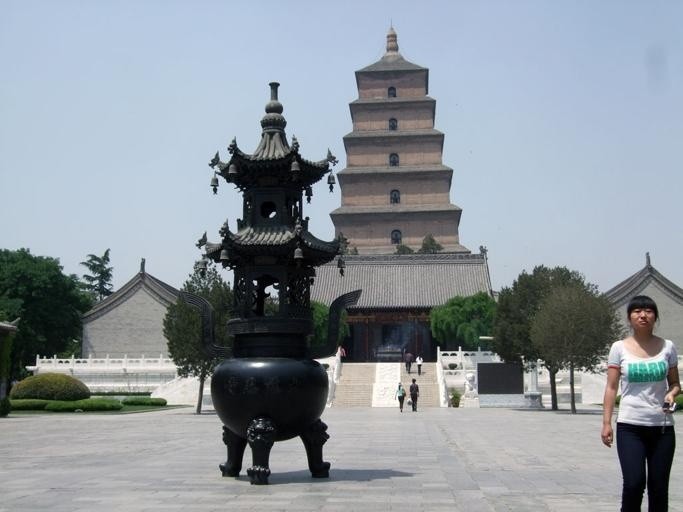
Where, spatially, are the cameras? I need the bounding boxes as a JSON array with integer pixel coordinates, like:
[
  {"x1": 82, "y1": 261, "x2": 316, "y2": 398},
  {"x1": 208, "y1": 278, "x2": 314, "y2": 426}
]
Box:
[{"x1": 662, "y1": 401, "x2": 678, "y2": 412}]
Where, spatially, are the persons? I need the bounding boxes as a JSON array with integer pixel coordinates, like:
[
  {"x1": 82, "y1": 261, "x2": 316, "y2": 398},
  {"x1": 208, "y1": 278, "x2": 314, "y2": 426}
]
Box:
[
  {"x1": 600, "y1": 295, "x2": 681, "y2": 512},
  {"x1": 394, "y1": 383, "x2": 406, "y2": 412},
  {"x1": 340, "y1": 346, "x2": 346, "y2": 362},
  {"x1": 415, "y1": 354, "x2": 423, "y2": 375},
  {"x1": 403, "y1": 351, "x2": 413, "y2": 374},
  {"x1": 409, "y1": 378, "x2": 419, "y2": 411}
]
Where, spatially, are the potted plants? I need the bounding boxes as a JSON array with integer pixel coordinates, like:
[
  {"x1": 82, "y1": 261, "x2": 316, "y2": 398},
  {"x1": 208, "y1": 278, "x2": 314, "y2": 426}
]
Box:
[{"x1": 451, "y1": 393, "x2": 459, "y2": 407}]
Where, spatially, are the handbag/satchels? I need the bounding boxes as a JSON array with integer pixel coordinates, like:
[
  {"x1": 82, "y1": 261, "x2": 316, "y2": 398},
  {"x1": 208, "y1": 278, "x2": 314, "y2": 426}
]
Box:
[
  {"x1": 402, "y1": 389, "x2": 406, "y2": 397},
  {"x1": 407, "y1": 398, "x2": 413, "y2": 405}
]
